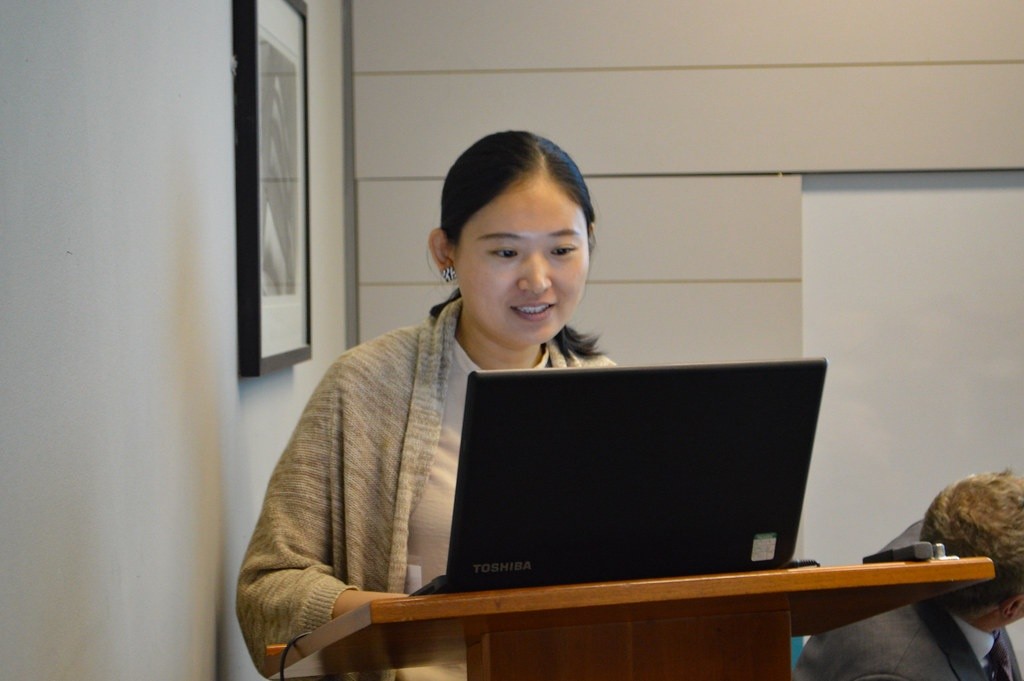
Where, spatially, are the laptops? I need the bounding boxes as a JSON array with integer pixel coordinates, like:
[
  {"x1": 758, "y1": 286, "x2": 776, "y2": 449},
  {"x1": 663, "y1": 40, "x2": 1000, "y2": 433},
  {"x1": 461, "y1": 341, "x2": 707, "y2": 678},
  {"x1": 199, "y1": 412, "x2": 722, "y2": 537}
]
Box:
[{"x1": 410, "y1": 356, "x2": 831, "y2": 596}]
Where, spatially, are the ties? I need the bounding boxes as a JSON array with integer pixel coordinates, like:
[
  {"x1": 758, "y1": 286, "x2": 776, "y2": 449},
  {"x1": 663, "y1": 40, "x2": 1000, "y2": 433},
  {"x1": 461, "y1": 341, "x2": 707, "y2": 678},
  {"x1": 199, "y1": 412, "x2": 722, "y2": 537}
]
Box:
[{"x1": 986, "y1": 629, "x2": 1013, "y2": 681}]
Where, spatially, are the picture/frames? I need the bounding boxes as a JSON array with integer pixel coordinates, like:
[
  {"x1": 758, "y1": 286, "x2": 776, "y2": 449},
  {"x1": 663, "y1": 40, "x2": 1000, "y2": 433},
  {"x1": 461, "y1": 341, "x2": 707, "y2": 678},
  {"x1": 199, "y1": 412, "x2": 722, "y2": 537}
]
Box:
[{"x1": 230, "y1": 1, "x2": 316, "y2": 377}]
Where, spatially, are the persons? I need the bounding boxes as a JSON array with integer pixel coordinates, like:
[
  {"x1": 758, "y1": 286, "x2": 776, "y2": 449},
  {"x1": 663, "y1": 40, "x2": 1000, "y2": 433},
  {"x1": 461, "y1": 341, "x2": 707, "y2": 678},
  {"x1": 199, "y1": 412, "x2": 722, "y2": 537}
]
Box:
[
  {"x1": 235, "y1": 130, "x2": 620, "y2": 681},
  {"x1": 791, "y1": 466, "x2": 1024, "y2": 681}
]
